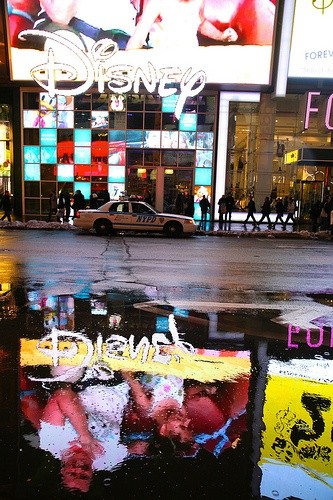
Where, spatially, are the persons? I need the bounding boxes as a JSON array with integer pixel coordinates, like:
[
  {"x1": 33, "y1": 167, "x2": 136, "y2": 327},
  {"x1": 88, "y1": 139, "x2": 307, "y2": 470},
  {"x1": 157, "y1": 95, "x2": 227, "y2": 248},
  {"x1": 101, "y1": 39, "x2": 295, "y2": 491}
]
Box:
[
  {"x1": 63, "y1": 192, "x2": 70, "y2": 222},
  {"x1": 198, "y1": 195, "x2": 210, "y2": 224},
  {"x1": 142, "y1": 190, "x2": 150, "y2": 205},
  {"x1": 56, "y1": 193, "x2": 64, "y2": 222},
  {"x1": 217, "y1": 195, "x2": 226, "y2": 223},
  {"x1": 70, "y1": 190, "x2": 109, "y2": 219},
  {"x1": 184, "y1": 195, "x2": 194, "y2": 218},
  {"x1": 224, "y1": 192, "x2": 234, "y2": 222},
  {"x1": 0, "y1": 191, "x2": 12, "y2": 223},
  {"x1": 272, "y1": 198, "x2": 285, "y2": 228},
  {"x1": 255, "y1": 197, "x2": 272, "y2": 226},
  {"x1": 282, "y1": 197, "x2": 297, "y2": 227},
  {"x1": 309, "y1": 194, "x2": 333, "y2": 236},
  {"x1": 32, "y1": 0, "x2": 142, "y2": 56},
  {"x1": 120, "y1": 369, "x2": 216, "y2": 455},
  {"x1": 241, "y1": 198, "x2": 256, "y2": 224},
  {"x1": 125, "y1": 0, "x2": 238, "y2": 51},
  {"x1": 37, "y1": 366, "x2": 129, "y2": 496},
  {"x1": 270, "y1": 189, "x2": 276, "y2": 201},
  {"x1": 45, "y1": 193, "x2": 60, "y2": 222},
  {"x1": 174, "y1": 194, "x2": 183, "y2": 214}
]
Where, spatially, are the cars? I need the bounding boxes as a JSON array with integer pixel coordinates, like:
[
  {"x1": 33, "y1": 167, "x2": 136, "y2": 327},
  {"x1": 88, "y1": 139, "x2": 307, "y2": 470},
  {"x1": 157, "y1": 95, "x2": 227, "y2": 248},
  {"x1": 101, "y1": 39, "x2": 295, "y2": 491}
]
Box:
[{"x1": 73, "y1": 195, "x2": 195, "y2": 238}]
